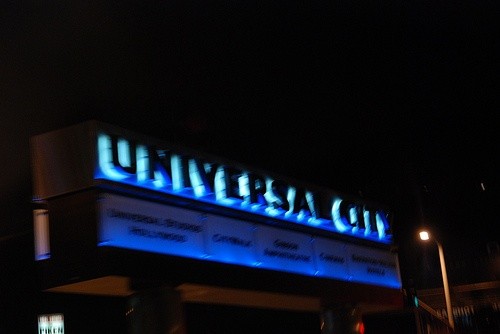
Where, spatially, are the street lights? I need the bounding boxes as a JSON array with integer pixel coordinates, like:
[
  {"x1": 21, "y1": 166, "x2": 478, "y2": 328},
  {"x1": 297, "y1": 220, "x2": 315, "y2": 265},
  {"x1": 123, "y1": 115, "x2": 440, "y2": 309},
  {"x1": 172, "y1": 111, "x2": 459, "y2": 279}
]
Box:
[{"x1": 419, "y1": 227, "x2": 454, "y2": 331}]
